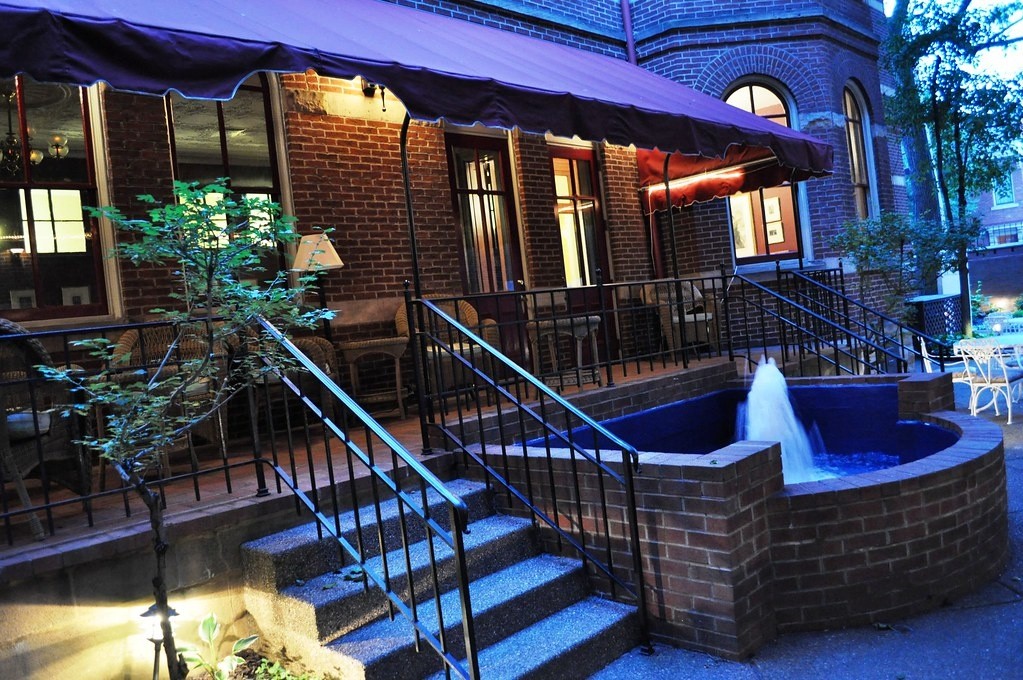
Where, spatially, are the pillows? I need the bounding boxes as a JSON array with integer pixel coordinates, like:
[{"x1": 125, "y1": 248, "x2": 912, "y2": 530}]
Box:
[{"x1": 426, "y1": 340, "x2": 484, "y2": 357}]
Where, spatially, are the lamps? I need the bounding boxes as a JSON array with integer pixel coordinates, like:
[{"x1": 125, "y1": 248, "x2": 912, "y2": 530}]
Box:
[
  {"x1": 0, "y1": 83, "x2": 71, "y2": 173},
  {"x1": 293, "y1": 234, "x2": 343, "y2": 344}
]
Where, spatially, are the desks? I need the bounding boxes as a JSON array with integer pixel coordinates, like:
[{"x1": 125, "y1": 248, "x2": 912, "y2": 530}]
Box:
[
  {"x1": 341, "y1": 338, "x2": 409, "y2": 423},
  {"x1": 954, "y1": 332, "x2": 1023, "y2": 403},
  {"x1": 526, "y1": 315, "x2": 603, "y2": 399}
]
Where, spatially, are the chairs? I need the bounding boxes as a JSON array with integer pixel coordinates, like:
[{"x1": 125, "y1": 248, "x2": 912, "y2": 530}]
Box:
[
  {"x1": 395, "y1": 293, "x2": 502, "y2": 406},
  {"x1": 919, "y1": 313, "x2": 1023, "y2": 424},
  {"x1": 1, "y1": 308, "x2": 339, "y2": 543},
  {"x1": 640, "y1": 278, "x2": 726, "y2": 363}
]
